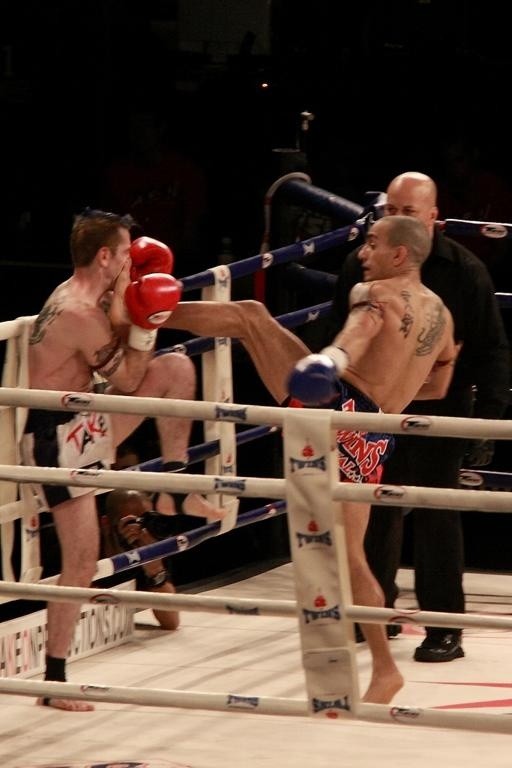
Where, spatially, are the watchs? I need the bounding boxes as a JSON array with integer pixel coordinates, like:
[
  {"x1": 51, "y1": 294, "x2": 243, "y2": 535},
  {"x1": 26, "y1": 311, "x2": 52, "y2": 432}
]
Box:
[{"x1": 144, "y1": 568, "x2": 168, "y2": 589}]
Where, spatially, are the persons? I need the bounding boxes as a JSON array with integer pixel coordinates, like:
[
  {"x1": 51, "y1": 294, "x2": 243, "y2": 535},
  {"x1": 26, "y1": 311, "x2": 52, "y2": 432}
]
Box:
[
  {"x1": 328, "y1": 165, "x2": 512, "y2": 664},
  {"x1": 109, "y1": 213, "x2": 458, "y2": 706},
  {"x1": 92, "y1": 487, "x2": 179, "y2": 632},
  {"x1": 107, "y1": 439, "x2": 143, "y2": 472},
  {"x1": 15, "y1": 208, "x2": 232, "y2": 714}
]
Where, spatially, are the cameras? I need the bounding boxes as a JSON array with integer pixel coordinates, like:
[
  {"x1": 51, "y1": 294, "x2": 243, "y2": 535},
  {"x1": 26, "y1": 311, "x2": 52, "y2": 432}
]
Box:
[{"x1": 127, "y1": 509, "x2": 207, "y2": 541}]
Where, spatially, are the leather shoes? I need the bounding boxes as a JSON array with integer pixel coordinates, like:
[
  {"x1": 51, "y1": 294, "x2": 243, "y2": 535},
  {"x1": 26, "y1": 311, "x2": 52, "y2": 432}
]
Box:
[
  {"x1": 353, "y1": 622, "x2": 402, "y2": 643},
  {"x1": 414, "y1": 634, "x2": 465, "y2": 662}
]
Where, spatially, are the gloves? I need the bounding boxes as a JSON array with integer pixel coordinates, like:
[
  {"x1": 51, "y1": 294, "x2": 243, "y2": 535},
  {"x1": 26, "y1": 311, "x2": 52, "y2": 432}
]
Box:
[
  {"x1": 123, "y1": 236, "x2": 184, "y2": 352},
  {"x1": 286, "y1": 344, "x2": 349, "y2": 405}
]
